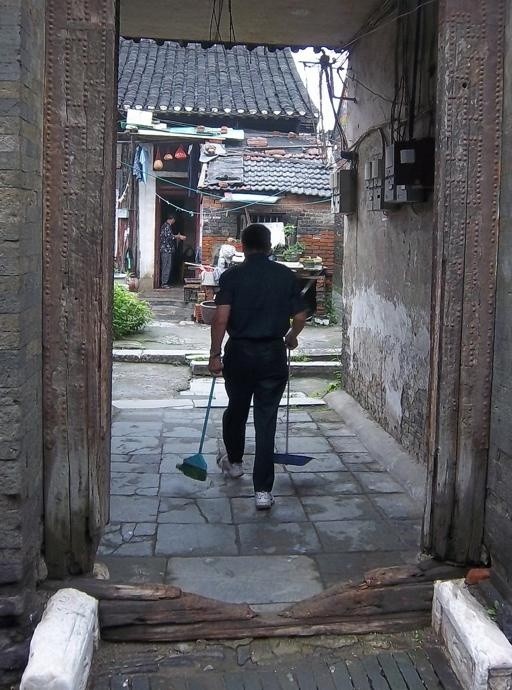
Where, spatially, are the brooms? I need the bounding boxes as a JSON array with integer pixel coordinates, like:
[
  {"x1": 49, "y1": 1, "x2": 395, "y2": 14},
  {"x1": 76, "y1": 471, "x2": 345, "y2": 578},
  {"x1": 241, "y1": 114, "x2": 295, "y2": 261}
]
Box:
[{"x1": 175, "y1": 372, "x2": 218, "y2": 481}]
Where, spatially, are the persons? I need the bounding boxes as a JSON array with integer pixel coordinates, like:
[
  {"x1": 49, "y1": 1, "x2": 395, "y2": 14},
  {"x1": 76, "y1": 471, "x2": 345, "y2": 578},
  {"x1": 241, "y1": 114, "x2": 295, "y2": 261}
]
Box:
[
  {"x1": 209, "y1": 224, "x2": 305, "y2": 510},
  {"x1": 159, "y1": 212, "x2": 187, "y2": 288}
]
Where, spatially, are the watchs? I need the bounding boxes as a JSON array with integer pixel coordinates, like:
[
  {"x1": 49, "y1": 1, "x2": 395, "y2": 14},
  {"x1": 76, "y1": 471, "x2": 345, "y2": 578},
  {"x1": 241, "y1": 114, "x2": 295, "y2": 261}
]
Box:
[{"x1": 210, "y1": 350, "x2": 221, "y2": 359}]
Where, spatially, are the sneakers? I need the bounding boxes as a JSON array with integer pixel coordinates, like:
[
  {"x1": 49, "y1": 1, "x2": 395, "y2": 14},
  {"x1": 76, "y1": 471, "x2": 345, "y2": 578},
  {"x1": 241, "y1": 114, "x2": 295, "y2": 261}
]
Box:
[
  {"x1": 216, "y1": 452, "x2": 244, "y2": 478},
  {"x1": 255, "y1": 490, "x2": 274, "y2": 509},
  {"x1": 161, "y1": 284, "x2": 174, "y2": 289}
]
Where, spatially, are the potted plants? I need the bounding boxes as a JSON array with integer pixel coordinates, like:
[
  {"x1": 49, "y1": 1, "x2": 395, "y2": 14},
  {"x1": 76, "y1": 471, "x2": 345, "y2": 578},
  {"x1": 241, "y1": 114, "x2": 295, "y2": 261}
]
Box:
[{"x1": 272, "y1": 225, "x2": 305, "y2": 263}]
